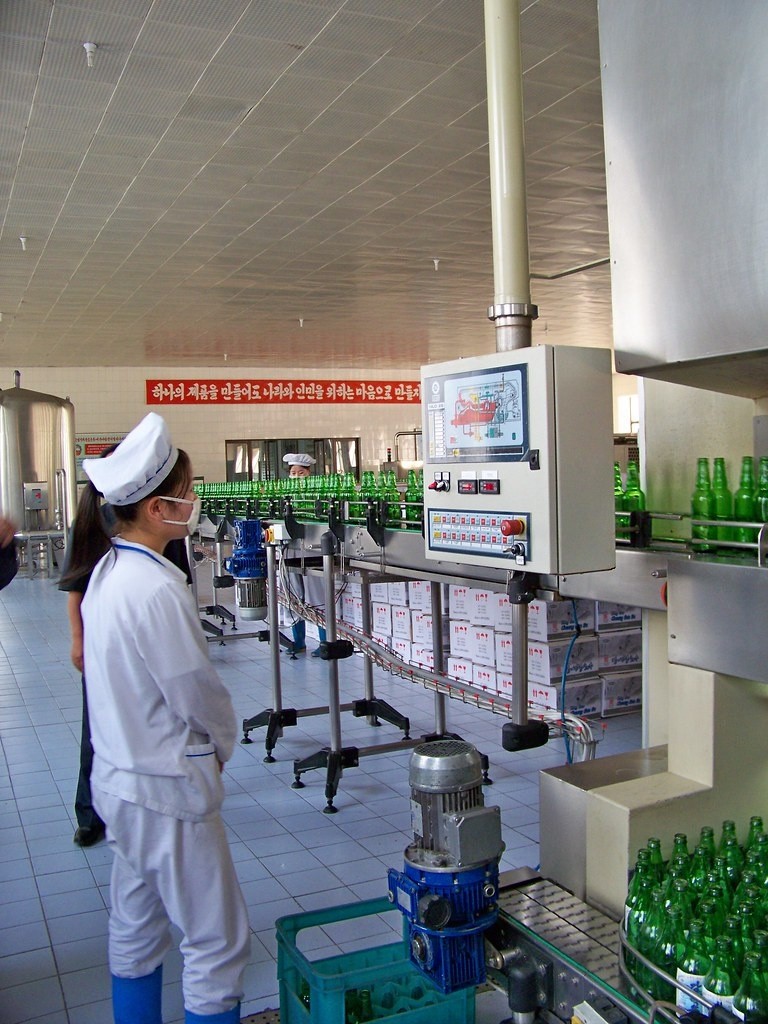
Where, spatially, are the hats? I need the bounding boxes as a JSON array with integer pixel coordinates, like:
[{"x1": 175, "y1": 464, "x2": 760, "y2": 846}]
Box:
[
  {"x1": 283, "y1": 452, "x2": 318, "y2": 467},
  {"x1": 81, "y1": 410, "x2": 181, "y2": 505}
]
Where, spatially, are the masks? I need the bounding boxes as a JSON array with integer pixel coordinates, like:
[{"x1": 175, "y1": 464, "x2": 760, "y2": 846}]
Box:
[{"x1": 158, "y1": 495, "x2": 200, "y2": 536}]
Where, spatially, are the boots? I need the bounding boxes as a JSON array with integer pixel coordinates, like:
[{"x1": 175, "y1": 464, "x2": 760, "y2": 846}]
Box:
[
  {"x1": 112, "y1": 964, "x2": 164, "y2": 1024},
  {"x1": 184, "y1": 1000, "x2": 242, "y2": 1024}
]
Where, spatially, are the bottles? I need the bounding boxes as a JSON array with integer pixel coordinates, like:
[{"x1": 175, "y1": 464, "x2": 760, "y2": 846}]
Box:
[
  {"x1": 690, "y1": 458, "x2": 717, "y2": 552},
  {"x1": 300, "y1": 978, "x2": 372, "y2": 1024},
  {"x1": 614, "y1": 461, "x2": 625, "y2": 546},
  {"x1": 752, "y1": 456, "x2": 768, "y2": 559},
  {"x1": 623, "y1": 461, "x2": 645, "y2": 546},
  {"x1": 735, "y1": 456, "x2": 758, "y2": 558},
  {"x1": 624, "y1": 816, "x2": 768, "y2": 1024},
  {"x1": 712, "y1": 457, "x2": 736, "y2": 557},
  {"x1": 191, "y1": 469, "x2": 423, "y2": 530}
]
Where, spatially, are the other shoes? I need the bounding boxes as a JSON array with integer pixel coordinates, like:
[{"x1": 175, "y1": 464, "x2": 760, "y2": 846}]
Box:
[
  {"x1": 312, "y1": 647, "x2": 321, "y2": 656},
  {"x1": 285, "y1": 646, "x2": 307, "y2": 655}
]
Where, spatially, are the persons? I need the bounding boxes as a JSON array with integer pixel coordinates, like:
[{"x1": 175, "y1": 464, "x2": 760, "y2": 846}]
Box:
[
  {"x1": 59, "y1": 444, "x2": 194, "y2": 846},
  {"x1": 82, "y1": 412, "x2": 251, "y2": 1024},
  {"x1": 283, "y1": 454, "x2": 317, "y2": 479}
]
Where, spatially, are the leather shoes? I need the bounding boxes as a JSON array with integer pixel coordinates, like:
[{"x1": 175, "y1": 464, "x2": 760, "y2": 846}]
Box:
[{"x1": 74, "y1": 826, "x2": 102, "y2": 846}]
